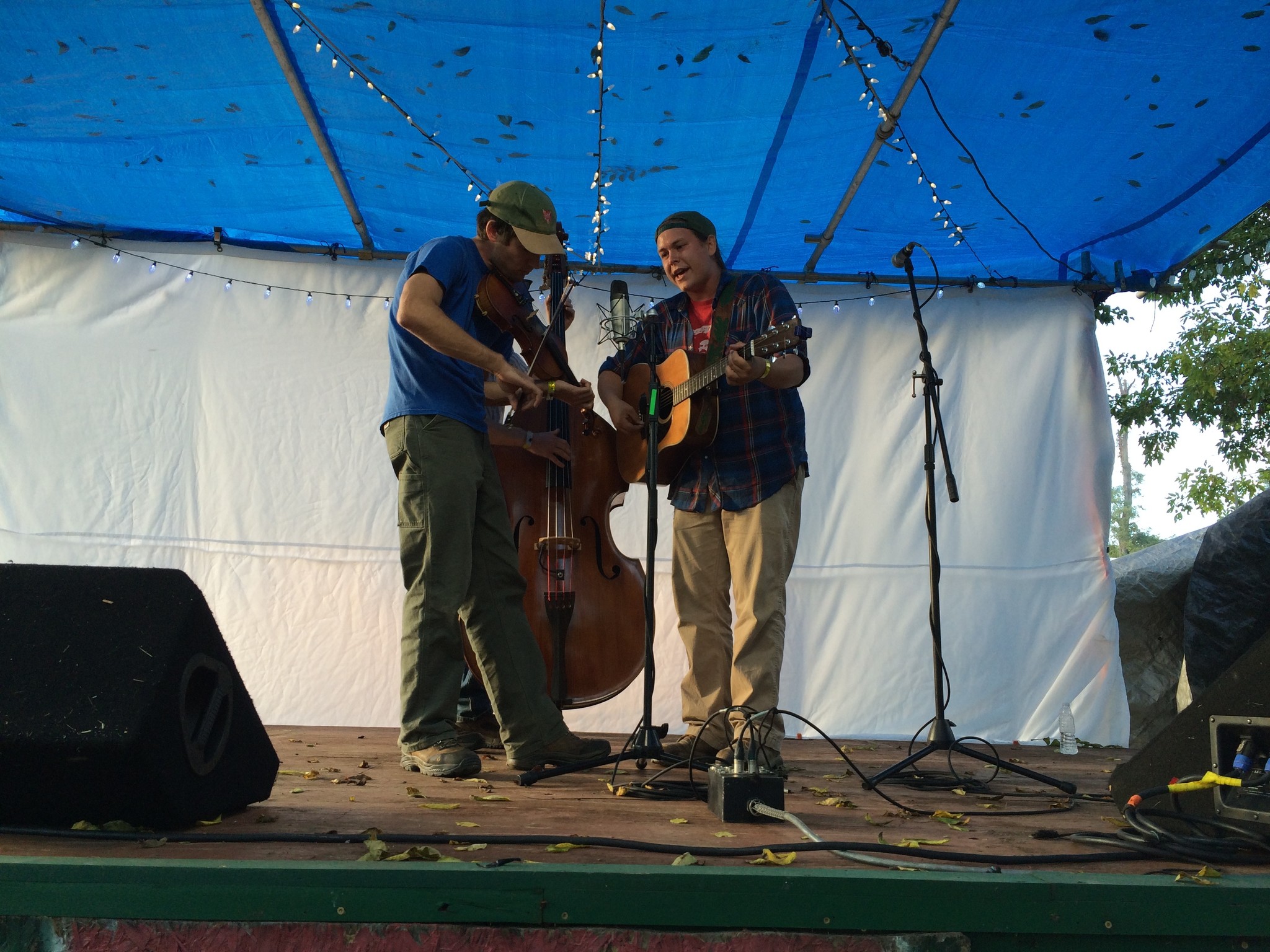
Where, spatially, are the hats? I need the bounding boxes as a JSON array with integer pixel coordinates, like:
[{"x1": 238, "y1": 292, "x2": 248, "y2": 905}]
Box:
[
  {"x1": 480, "y1": 181, "x2": 567, "y2": 254},
  {"x1": 655, "y1": 211, "x2": 717, "y2": 241}
]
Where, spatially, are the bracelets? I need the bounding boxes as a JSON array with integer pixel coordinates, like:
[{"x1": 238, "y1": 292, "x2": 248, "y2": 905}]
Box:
[
  {"x1": 757, "y1": 357, "x2": 771, "y2": 381},
  {"x1": 521, "y1": 431, "x2": 535, "y2": 450},
  {"x1": 546, "y1": 379, "x2": 556, "y2": 401}
]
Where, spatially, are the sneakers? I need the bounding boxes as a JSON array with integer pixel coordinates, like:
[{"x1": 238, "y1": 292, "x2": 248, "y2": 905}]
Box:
[
  {"x1": 506, "y1": 729, "x2": 610, "y2": 770},
  {"x1": 716, "y1": 740, "x2": 784, "y2": 775},
  {"x1": 401, "y1": 740, "x2": 481, "y2": 776},
  {"x1": 651, "y1": 733, "x2": 717, "y2": 768}
]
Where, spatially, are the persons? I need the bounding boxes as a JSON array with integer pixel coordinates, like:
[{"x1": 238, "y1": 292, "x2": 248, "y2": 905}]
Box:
[
  {"x1": 597, "y1": 212, "x2": 810, "y2": 773},
  {"x1": 380, "y1": 179, "x2": 612, "y2": 775}
]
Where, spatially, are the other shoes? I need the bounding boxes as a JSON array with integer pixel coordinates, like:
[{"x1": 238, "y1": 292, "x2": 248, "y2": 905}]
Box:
[{"x1": 455, "y1": 725, "x2": 504, "y2": 748}]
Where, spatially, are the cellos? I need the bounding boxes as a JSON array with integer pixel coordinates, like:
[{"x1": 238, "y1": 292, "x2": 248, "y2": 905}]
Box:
[{"x1": 462, "y1": 225, "x2": 657, "y2": 712}]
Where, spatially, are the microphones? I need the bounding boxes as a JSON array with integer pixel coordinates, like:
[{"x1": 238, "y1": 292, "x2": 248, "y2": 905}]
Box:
[
  {"x1": 610, "y1": 280, "x2": 629, "y2": 363},
  {"x1": 892, "y1": 243, "x2": 915, "y2": 267}
]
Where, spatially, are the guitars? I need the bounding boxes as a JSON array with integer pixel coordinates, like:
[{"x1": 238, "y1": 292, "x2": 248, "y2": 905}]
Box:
[{"x1": 616, "y1": 316, "x2": 813, "y2": 487}]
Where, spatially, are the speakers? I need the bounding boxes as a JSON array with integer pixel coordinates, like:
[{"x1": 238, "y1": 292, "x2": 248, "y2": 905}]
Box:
[
  {"x1": 0, "y1": 564, "x2": 280, "y2": 828},
  {"x1": 1108, "y1": 630, "x2": 1270, "y2": 849}
]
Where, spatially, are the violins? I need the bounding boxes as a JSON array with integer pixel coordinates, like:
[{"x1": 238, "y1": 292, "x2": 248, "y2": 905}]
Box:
[{"x1": 474, "y1": 270, "x2": 602, "y2": 438}]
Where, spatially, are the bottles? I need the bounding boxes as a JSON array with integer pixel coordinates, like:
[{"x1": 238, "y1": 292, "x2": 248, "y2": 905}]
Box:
[{"x1": 1058, "y1": 703, "x2": 1078, "y2": 754}]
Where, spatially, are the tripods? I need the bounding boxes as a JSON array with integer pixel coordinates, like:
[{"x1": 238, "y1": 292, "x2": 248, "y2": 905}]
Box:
[
  {"x1": 513, "y1": 309, "x2": 710, "y2": 787},
  {"x1": 862, "y1": 255, "x2": 1078, "y2": 794}
]
